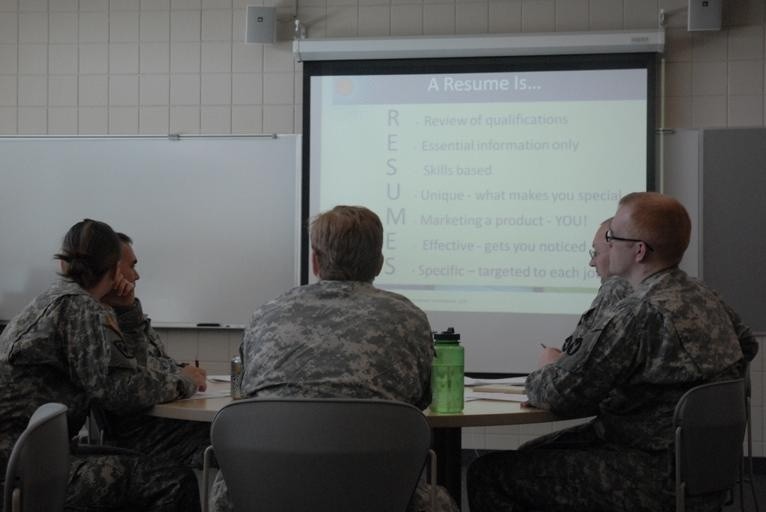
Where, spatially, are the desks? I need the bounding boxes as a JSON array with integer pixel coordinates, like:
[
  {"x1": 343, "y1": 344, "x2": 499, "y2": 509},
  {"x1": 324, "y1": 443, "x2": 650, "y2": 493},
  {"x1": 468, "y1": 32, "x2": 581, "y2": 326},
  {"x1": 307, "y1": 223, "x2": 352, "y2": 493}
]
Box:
[{"x1": 148, "y1": 374, "x2": 598, "y2": 509}]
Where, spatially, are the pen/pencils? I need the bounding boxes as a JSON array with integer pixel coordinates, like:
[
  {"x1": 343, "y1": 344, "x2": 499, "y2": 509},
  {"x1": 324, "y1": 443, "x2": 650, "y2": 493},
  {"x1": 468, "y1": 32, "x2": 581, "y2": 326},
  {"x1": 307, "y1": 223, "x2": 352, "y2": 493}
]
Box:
[
  {"x1": 540, "y1": 343, "x2": 546, "y2": 349},
  {"x1": 194, "y1": 358, "x2": 200, "y2": 368}
]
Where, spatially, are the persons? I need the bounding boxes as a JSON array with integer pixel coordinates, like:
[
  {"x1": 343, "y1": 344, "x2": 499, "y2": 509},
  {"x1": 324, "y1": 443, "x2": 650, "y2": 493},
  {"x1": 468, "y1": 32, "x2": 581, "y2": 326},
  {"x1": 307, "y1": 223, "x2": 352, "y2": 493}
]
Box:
[
  {"x1": 92, "y1": 231, "x2": 213, "y2": 467},
  {"x1": 560, "y1": 215, "x2": 761, "y2": 431},
  {"x1": 0, "y1": 219, "x2": 207, "y2": 510},
  {"x1": 210, "y1": 203, "x2": 460, "y2": 509},
  {"x1": 474, "y1": 191, "x2": 746, "y2": 511}
]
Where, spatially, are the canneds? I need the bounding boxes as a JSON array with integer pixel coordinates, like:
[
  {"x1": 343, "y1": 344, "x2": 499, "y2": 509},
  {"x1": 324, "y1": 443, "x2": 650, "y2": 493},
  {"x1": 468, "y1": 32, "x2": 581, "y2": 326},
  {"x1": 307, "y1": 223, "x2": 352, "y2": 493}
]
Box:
[{"x1": 231, "y1": 357, "x2": 242, "y2": 400}]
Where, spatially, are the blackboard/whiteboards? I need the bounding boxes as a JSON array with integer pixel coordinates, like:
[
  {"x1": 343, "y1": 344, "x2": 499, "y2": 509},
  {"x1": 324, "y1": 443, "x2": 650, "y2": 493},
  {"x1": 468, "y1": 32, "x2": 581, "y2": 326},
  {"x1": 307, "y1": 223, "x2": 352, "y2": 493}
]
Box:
[{"x1": 0, "y1": 133, "x2": 303, "y2": 330}]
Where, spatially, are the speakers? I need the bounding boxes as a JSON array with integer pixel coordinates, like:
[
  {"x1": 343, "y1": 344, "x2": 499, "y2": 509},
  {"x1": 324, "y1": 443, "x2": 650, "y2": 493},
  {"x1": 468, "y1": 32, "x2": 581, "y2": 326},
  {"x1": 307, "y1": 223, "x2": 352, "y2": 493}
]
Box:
[
  {"x1": 245, "y1": 7, "x2": 277, "y2": 45},
  {"x1": 687, "y1": 0, "x2": 722, "y2": 31}
]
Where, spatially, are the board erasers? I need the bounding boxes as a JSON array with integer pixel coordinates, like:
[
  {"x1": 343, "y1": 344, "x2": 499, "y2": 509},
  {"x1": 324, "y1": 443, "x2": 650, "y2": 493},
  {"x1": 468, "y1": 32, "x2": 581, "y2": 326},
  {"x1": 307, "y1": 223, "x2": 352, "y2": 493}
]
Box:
[{"x1": 197, "y1": 323, "x2": 221, "y2": 326}]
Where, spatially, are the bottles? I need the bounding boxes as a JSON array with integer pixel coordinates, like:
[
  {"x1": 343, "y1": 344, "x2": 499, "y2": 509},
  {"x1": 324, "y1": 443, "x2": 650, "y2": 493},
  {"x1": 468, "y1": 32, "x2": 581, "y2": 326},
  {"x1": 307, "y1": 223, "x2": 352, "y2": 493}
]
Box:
[{"x1": 429, "y1": 328, "x2": 464, "y2": 413}]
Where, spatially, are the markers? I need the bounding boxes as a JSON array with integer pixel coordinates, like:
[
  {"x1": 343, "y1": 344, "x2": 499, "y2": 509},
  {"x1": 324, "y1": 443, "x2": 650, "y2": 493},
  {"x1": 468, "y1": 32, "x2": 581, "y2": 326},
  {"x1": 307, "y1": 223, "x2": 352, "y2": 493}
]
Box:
[{"x1": 225, "y1": 324, "x2": 243, "y2": 328}]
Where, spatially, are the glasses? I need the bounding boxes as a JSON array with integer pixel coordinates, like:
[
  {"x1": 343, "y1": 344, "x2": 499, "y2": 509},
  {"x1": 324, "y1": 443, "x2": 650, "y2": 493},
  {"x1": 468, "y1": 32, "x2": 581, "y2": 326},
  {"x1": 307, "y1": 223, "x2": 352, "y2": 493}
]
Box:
[
  {"x1": 589, "y1": 249, "x2": 598, "y2": 258},
  {"x1": 606, "y1": 231, "x2": 654, "y2": 252}
]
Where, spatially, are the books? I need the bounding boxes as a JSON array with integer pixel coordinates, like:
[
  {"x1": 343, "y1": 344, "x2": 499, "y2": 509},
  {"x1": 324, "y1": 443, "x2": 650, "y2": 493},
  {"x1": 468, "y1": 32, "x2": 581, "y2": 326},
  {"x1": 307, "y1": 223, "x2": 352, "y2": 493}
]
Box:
[{"x1": 475, "y1": 383, "x2": 527, "y2": 396}]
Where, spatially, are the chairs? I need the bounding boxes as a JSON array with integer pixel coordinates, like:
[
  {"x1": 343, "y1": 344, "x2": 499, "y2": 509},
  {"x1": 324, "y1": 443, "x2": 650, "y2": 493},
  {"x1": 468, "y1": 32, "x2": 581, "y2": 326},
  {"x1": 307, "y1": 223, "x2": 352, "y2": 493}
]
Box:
[
  {"x1": 0, "y1": 402, "x2": 70, "y2": 510},
  {"x1": 671, "y1": 377, "x2": 749, "y2": 512},
  {"x1": 735, "y1": 359, "x2": 761, "y2": 512},
  {"x1": 199, "y1": 396, "x2": 440, "y2": 509}
]
